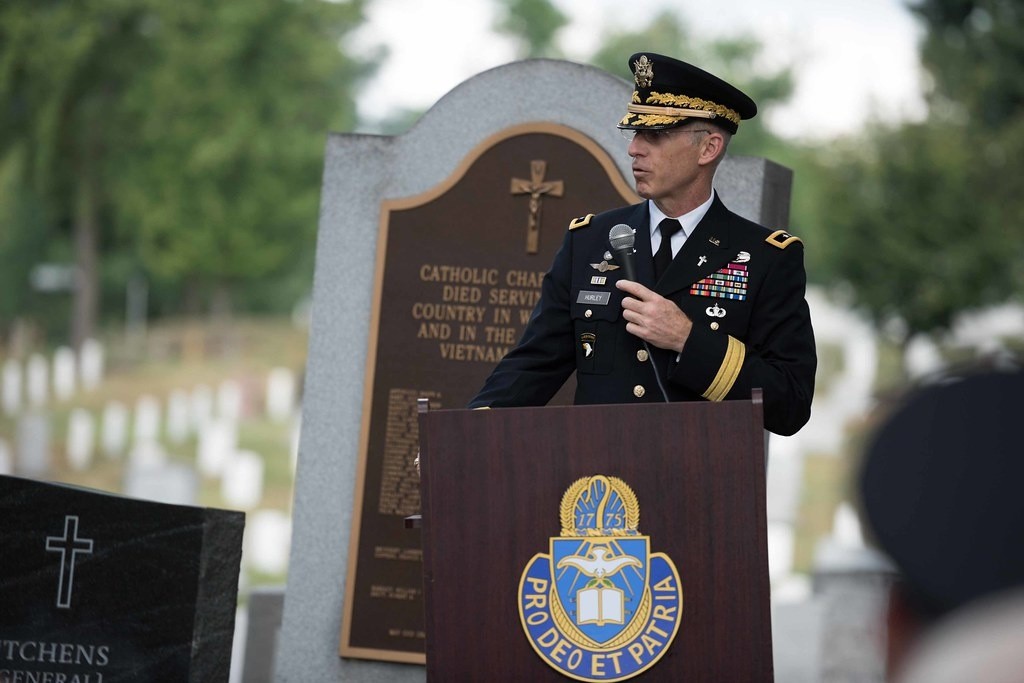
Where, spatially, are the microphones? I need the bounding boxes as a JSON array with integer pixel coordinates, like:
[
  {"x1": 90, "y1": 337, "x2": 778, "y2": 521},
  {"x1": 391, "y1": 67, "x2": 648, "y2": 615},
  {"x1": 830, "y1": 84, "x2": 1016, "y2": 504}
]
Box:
[{"x1": 609, "y1": 224, "x2": 638, "y2": 301}]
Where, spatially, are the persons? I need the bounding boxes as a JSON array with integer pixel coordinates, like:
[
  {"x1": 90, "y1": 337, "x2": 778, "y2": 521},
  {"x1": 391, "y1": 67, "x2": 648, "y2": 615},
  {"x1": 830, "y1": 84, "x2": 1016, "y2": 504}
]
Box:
[
  {"x1": 468, "y1": 53, "x2": 818, "y2": 436},
  {"x1": 853, "y1": 362, "x2": 1024, "y2": 683}
]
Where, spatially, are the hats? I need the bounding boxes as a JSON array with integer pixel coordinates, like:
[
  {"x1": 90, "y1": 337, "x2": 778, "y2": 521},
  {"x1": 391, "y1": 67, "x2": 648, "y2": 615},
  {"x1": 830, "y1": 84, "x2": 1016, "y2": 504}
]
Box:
[{"x1": 617, "y1": 52, "x2": 758, "y2": 135}]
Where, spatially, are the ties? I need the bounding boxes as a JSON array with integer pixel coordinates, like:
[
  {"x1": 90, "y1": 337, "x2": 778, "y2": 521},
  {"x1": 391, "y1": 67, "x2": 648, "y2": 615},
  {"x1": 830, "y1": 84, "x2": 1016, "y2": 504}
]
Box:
[{"x1": 652, "y1": 219, "x2": 682, "y2": 283}]
